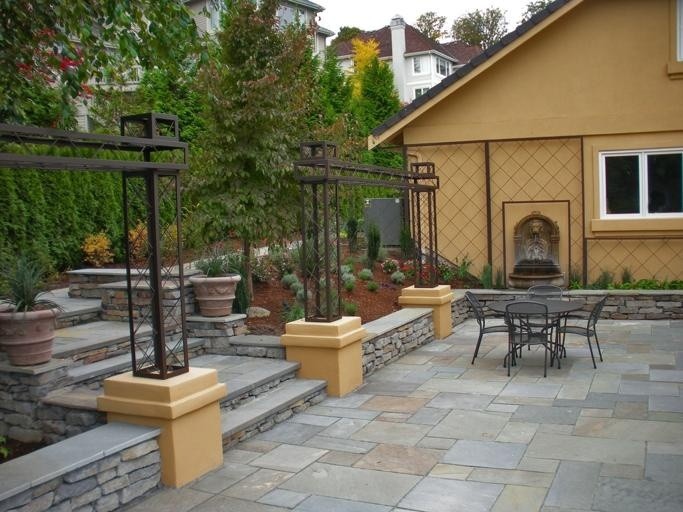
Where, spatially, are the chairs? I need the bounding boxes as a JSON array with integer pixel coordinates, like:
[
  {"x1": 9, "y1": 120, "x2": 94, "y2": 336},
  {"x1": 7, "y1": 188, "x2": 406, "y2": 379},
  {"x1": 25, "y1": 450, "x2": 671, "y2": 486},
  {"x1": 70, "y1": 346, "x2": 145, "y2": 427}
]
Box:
[{"x1": 464, "y1": 283, "x2": 611, "y2": 378}]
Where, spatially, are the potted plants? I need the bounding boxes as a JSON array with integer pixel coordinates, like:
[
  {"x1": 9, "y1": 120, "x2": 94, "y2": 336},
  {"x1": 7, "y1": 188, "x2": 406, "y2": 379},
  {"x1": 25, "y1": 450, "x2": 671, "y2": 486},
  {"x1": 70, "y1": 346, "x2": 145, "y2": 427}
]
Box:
[
  {"x1": 0, "y1": 255, "x2": 65, "y2": 367},
  {"x1": 188, "y1": 251, "x2": 241, "y2": 316}
]
